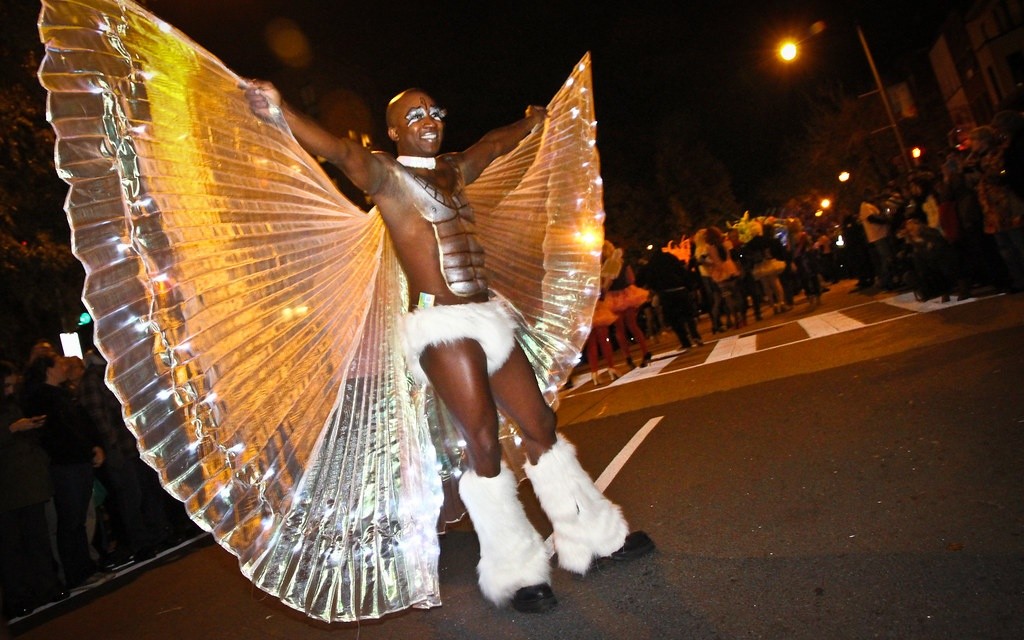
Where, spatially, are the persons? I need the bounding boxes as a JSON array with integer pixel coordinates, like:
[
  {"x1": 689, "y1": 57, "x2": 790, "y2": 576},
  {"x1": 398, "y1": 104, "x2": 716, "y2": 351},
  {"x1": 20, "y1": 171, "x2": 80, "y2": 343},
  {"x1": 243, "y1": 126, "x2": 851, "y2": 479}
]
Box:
[
  {"x1": 236, "y1": 78, "x2": 655, "y2": 612},
  {"x1": 558, "y1": 111, "x2": 1024, "y2": 385},
  {"x1": 859, "y1": 185, "x2": 906, "y2": 293},
  {"x1": 635, "y1": 238, "x2": 702, "y2": 350},
  {"x1": 0, "y1": 340, "x2": 204, "y2": 627}
]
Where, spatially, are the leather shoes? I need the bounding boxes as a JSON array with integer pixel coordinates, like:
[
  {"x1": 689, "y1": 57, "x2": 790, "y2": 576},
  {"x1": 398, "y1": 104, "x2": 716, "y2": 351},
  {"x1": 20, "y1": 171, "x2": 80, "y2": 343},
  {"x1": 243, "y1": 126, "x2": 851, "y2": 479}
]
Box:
[
  {"x1": 511, "y1": 583, "x2": 557, "y2": 611},
  {"x1": 574, "y1": 531, "x2": 653, "y2": 576}
]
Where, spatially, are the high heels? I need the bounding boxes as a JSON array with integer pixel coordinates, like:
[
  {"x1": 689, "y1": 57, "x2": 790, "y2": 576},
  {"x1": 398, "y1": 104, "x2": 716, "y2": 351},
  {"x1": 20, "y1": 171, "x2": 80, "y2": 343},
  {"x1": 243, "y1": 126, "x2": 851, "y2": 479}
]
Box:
[
  {"x1": 608, "y1": 368, "x2": 621, "y2": 382},
  {"x1": 592, "y1": 371, "x2": 603, "y2": 387},
  {"x1": 639, "y1": 352, "x2": 652, "y2": 367},
  {"x1": 627, "y1": 357, "x2": 635, "y2": 371}
]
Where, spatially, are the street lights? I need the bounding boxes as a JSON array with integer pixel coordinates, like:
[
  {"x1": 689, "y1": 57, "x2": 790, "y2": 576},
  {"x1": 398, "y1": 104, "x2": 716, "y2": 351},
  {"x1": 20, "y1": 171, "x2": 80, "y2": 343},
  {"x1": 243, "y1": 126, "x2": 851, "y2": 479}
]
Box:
[{"x1": 779, "y1": 10, "x2": 911, "y2": 173}]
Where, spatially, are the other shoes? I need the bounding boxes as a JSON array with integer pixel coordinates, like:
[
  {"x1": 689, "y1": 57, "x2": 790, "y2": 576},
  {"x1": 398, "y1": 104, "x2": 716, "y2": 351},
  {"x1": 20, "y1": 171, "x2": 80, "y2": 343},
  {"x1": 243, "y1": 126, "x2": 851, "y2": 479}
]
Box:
[
  {"x1": 940, "y1": 290, "x2": 950, "y2": 303},
  {"x1": 136, "y1": 545, "x2": 154, "y2": 561},
  {"x1": 694, "y1": 337, "x2": 703, "y2": 345},
  {"x1": 48, "y1": 580, "x2": 66, "y2": 600},
  {"x1": 158, "y1": 536, "x2": 182, "y2": 549},
  {"x1": 780, "y1": 305, "x2": 792, "y2": 312},
  {"x1": 17, "y1": 594, "x2": 33, "y2": 617},
  {"x1": 678, "y1": 344, "x2": 690, "y2": 350},
  {"x1": 68, "y1": 571, "x2": 117, "y2": 592},
  {"x1": 773, "y1": 307, "x2": 779, "y2": 314},
  {"x1": 959, "y1": 288, "x2": 970, "y2": 301}
]
